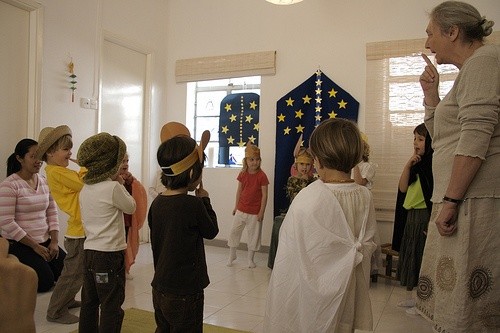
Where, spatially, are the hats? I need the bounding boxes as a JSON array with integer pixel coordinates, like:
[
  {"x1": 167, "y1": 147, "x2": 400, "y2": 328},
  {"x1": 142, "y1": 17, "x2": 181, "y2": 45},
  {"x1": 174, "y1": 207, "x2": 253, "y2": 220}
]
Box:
[
  {"x1": 77, "y1": 131, "x2": 127, "y2": 183},
  {"x1": 33, "y1": 125, "x2": 72, "y2": 161}
]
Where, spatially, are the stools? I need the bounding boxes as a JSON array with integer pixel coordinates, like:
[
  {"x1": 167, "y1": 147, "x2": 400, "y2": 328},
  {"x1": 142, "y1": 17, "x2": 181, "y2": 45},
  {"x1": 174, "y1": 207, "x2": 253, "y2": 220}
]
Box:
[{"x1": 372, "y1": 243, "x2": 400, "y2": 283}]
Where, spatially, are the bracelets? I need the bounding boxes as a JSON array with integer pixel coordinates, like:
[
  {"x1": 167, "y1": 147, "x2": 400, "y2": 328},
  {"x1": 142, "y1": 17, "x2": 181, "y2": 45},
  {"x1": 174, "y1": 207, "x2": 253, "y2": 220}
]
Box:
[{"x1": 443, "y1": 195, "x2": 463, "y2": 204}]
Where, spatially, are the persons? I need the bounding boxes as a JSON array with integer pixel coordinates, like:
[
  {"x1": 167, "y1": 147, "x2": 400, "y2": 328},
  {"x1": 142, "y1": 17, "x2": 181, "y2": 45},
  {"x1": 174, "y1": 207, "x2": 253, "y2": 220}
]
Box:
[
  {"x1": 148, "y1": 170, "x2": 167, "y2": 199},
  {"x1": 226, "y1": 146, "x2": 269, "y2": 267},
  {"x1": 415, "y1": 0, "x2": 500, "y2": 333},
  {"x1": 352, "y1": 140, "x2": 381, "y2": 274},
  {"x1": 392, "y1": 123, "x2": 433, "y2": 290},
  {"x1": 120, "y1": 155, "x2": 148, "y2": 279},
  {"x1": 34, "y1": 124, "x2": 89, "y2": 324},
  {"x1": 264, "y1": 118, "x2": 377, "y2": 333},
  {"x1": 147, "y1": 122, "x2": 219, "y2": 333},
  {"x1": 287, "y1": 148, "x2": 315, "y2": 204},
  {"x1": 77, "y1": 132, "x2": 136, "y2": 333},
  {"x1": 0, "y1": 138, "x2": 67, "y2": 292}
]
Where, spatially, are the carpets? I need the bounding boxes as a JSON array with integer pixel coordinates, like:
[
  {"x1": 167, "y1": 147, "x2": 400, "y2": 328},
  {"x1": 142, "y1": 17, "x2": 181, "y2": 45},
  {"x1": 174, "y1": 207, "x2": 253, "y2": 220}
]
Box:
[{"x1": 71, "y1": 308, "x2": 257, "y2": 333}]
